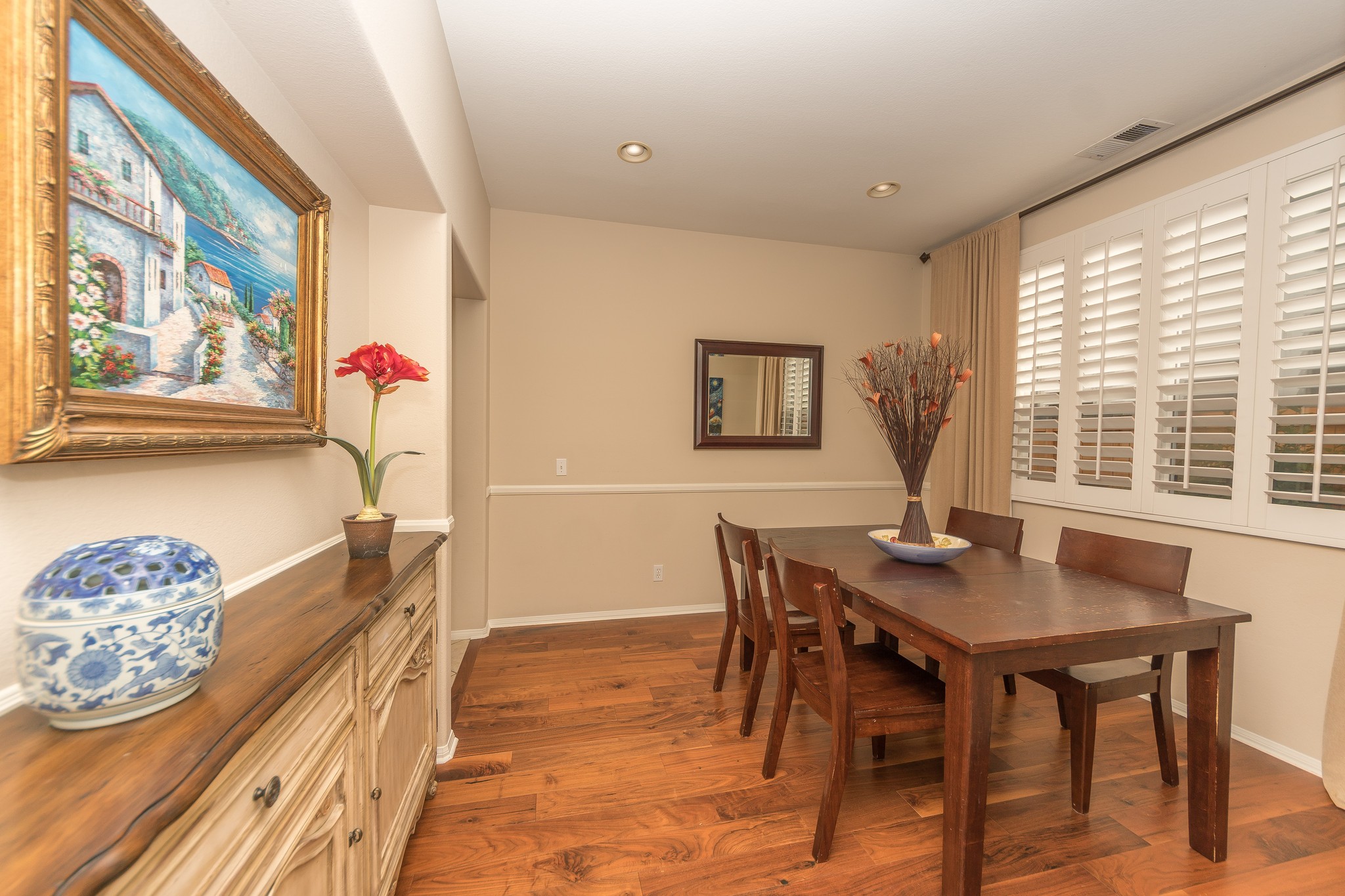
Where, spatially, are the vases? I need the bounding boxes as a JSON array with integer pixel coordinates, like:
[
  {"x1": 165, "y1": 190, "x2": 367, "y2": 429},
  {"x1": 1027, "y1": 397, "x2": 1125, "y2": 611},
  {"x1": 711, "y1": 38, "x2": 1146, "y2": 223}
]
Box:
[{"x1": 341, "y1": 513, "x2": 398, "y2": 560}]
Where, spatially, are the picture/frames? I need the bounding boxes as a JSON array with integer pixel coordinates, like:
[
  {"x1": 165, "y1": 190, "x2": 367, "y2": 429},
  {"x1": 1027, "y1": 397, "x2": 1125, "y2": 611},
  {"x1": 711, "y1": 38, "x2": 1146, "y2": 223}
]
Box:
[{"x1": 0, "y1": 0, "x2": 331, "y2": 463}]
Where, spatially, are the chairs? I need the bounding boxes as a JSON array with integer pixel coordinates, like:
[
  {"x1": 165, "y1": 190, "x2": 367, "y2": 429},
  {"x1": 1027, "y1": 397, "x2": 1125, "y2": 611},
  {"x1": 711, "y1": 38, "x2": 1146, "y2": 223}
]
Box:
[
  {"x1": 1018, "y1": 527, "x2": 1193, "y2": 816},
  {"x1": 761, "y1": 535, "x2": 946, "y2": 865},
  {"x1": 886, "y1": 505, "x2": 1024, "y2": 696},
  {"x1": 711, "y1": 512, "x2": 856, "y2": 736}
]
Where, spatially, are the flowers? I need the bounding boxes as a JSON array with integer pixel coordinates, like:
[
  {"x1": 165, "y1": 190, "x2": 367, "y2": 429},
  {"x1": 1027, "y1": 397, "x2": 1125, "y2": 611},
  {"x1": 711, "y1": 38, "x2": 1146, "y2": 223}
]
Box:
[{"x1": 311, "y1": 339, "x2": 430, "y2": 521}]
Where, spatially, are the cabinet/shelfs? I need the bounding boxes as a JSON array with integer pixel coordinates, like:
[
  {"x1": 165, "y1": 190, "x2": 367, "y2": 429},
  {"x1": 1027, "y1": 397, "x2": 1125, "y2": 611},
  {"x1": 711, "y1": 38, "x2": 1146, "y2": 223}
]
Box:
[{"x1": 0, "y1": 530, "x2": 448, "y2": 895}]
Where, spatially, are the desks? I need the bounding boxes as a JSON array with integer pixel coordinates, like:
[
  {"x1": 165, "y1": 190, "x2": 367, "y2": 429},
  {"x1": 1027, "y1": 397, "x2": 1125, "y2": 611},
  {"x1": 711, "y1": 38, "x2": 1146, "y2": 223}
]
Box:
[{"x1": 740, "y1": 523, "x2": 1252, "y2": 896}]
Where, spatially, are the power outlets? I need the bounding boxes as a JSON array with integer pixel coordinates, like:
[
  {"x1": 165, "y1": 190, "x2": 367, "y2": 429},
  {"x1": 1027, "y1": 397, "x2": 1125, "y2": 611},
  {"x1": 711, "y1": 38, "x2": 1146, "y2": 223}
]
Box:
[{"x1": 653, "y1": 565, "x2": 663, "y2": 581}]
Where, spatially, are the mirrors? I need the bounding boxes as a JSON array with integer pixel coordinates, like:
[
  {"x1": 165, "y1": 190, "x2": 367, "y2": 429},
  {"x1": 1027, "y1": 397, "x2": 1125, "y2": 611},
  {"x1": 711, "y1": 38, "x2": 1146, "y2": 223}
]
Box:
[{"x1": 695, "y1": 338, "x2": 823, "y2": 449}]
